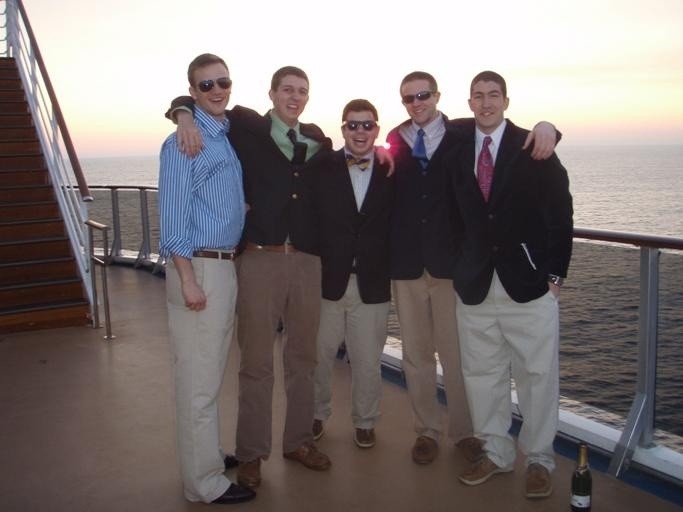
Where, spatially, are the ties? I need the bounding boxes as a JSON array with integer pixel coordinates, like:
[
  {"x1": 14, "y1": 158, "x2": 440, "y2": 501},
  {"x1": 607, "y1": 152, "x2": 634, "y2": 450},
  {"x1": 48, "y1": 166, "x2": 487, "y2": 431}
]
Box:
[
  {"x1": 477, "y1": 136, "x2": 494, "y2": 204},
  {"x1": 287, "y1": 128, "x2": 307, "y2": 167},
  {"x1": 411, "y1": 129, "x2": 430, "y2": 168}
]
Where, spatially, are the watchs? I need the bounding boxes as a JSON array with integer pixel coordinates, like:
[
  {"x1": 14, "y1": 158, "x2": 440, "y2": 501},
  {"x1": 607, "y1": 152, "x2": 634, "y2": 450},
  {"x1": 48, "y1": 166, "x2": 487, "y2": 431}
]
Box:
[{"x1": 548, "y1": 275, "x2": 563, "y2": 288}]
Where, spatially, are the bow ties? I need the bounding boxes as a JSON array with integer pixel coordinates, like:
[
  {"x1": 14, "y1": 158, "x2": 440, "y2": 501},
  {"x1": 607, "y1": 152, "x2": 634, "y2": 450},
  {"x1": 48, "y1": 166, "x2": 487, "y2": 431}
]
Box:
[{"x1": 346, "y1": 153, "x2": 370, "y2": 172}]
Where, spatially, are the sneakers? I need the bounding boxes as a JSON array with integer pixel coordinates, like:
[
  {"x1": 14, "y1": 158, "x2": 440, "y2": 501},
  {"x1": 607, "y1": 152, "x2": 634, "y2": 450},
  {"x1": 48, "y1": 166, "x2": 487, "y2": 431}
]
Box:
[
  {"x1": 312, "y1": 418, "x2": 325, "y2": 441},
  {"x1": 236, "y1": 456, "x2": 261, "y2": 488},
  {"x1": 283, "y1": 440, "x2": 332, "y2": 471},
  {"x1": 353, "y1": 426, "x2": 376, "y2": 449},
  {"x1": 456, "y1": 437, "x2": 515, "y2": 486},
  {"x1": 413, "y1": 435, "x2": 438, "y2": 465},
  {"x1": 525, "y1": 462, "x2": 554, "y2": 498}
]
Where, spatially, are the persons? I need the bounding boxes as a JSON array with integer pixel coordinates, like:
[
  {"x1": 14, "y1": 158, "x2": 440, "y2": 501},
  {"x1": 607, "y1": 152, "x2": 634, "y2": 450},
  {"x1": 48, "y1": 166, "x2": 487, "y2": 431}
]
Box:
[
  {"x1": 156, "y1": 54, "x2": 256, "y2": 503},
  {"x1": 390, "y1": 70, "x2": 563, "y2": 463},
  {"x1": 165, "y1": 66, "x2": 396, "y2": 490},
  {"x1": 443, "y1": 71, "x2": 574, "y2": 500},
  {"x1": 313, "y1": 98, "x2": 400, "y2": 449}
]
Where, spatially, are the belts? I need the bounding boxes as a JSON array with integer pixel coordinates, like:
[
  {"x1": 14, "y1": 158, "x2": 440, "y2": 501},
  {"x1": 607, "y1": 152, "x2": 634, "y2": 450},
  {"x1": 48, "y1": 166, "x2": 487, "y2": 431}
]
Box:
[
  {"x1": 193, "y1": 251, "x2": 237, "y2": 261},
  {"x1": 245, "y1": 241, "x2": 303, "y2": 256}
]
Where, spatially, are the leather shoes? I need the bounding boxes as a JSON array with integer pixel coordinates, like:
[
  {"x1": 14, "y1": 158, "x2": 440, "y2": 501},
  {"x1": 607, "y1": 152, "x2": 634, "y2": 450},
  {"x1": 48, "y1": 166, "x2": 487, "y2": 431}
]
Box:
[
  {"x1": 211, "y1": 483, "x2": 256, "y2": 504},
  {"x1": 226, "y1": 453, "x2": 239, "y2": 469}
]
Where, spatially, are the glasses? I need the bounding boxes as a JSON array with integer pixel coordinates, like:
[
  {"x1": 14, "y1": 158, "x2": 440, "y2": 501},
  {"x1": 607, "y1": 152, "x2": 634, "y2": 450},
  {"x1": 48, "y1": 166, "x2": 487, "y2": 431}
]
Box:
[
  {"x1": 341, "y1": 120, "x2": 379, "y2": 131},
  {"x1": 402, "y1": 91, "x2": 434, "y2": 105},
  {"x1": 198, "y1": 78, "x2": 232, "y2": 91}
]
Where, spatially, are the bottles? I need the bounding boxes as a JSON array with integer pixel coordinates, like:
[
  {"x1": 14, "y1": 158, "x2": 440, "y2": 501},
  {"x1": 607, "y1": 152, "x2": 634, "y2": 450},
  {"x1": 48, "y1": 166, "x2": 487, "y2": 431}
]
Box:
[{"x1": 571, "y1": 442, "x2": 593, "y2": 511}]
